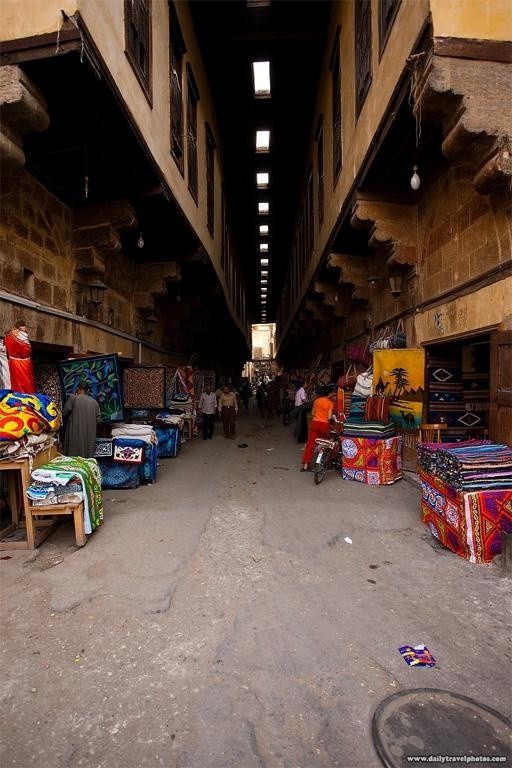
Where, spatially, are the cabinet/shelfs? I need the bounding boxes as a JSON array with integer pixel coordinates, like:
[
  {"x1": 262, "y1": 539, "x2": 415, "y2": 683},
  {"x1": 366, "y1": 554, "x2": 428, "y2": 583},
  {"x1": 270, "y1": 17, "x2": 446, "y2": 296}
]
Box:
[{"x1": 0, "y1": 437, "x2": 89, "y2": 551}]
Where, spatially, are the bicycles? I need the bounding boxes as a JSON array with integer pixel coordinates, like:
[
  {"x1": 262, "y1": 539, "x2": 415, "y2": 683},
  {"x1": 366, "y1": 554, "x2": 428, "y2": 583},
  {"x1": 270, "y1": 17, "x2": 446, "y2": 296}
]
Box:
[{"x1": 314, "y1": 419, "x2": 345, "y2": 484}]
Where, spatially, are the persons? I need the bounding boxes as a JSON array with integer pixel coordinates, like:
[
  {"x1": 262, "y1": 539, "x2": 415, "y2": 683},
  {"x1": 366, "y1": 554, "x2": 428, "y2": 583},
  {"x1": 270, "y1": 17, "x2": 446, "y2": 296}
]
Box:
[
  {"x1": 13, "y1": 319, "x2": 28, "y2": 333},
  {"x1": 62, "y1": 380, "x2": 101, "y2": 459},
  {"x1": 194, "y1": 373, "x2": 337, "y2": 472}
]
